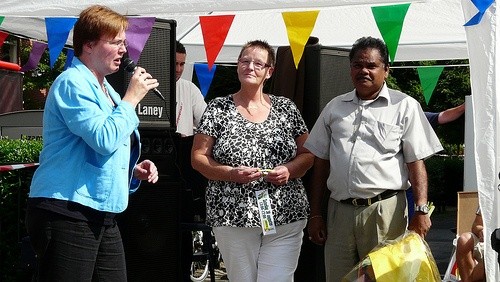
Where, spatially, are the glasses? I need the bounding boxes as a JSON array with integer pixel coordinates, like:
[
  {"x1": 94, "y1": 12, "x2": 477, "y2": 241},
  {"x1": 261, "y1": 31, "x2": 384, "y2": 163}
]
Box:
[
  {"x1": 239, "y1": 58, "x2": 269, "y2": 69},
  {"x1": 96, "y1": 39, "x2": 129, "y2": 47}
]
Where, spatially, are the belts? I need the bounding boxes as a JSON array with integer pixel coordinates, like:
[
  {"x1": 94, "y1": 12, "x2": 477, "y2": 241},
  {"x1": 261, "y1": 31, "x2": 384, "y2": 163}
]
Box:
[{"x1": 342, "y1": 189, "x2": 398, "y2": 206}]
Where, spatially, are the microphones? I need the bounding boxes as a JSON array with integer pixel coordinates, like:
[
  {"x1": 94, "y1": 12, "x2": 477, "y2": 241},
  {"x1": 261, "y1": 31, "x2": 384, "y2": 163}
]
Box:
[{"x1": 121, "y1": 57, "x2": 165, "y2": 100}]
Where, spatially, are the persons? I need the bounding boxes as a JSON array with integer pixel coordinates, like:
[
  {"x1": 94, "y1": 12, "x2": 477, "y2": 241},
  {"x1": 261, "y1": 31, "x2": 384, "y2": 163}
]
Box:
[
  {"x1": 25, "y1": 6, "x2": 160, "y2": 282},
  {"x1": 303, "y1": 37, "x2": 444, "y2": 282},
  {"x1": 404, "y1": 102, "x2": 465, "y2": 225},
  {"x1": 192, "y1": 41, "x2": 315, "y2": 282},
  {"x1": 457, "y1": 204, "x2": 485, "y2": 282},
  {"x1": 174, "y1": 41, "x2": 209, "y2": 282}
]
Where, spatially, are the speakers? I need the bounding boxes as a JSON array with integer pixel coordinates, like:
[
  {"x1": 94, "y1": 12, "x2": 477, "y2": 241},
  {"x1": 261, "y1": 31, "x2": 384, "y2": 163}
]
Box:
[
  {"x1": 102, "y1": 17, "x2": 178, "y2": 128},
  {"x1": 302, "y1": 44, "x2": 356, "y2": 135},
  {"x1": 115, "y1": 153, "x2": 184, "y2": 282}
]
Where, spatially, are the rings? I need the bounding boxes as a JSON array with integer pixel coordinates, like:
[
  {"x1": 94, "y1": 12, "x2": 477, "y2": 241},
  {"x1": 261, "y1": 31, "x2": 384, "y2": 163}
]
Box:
[
  {"x1": 280, "y1": 180, "x2": 282, "y2": 184},
  {"x1": 309, "y1": 236, "x2": 311, "y2": 239}
]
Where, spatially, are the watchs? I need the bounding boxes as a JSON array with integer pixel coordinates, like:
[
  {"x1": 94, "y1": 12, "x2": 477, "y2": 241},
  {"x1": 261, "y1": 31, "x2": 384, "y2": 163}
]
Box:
[{"x1": 414, "y1": 204, "x2": 428, "y2": 215}]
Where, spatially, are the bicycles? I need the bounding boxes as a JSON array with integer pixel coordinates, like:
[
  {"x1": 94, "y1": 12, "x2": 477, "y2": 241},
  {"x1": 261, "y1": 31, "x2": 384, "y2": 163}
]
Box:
[{"x1": 189, "y1": 230, "x2": 225, "y2": 282}]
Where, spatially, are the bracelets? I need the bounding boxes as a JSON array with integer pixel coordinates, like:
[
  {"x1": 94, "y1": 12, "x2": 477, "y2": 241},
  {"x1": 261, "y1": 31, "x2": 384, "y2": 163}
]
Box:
[{"x1": 308, "y1": 216, "x2": 324, "y2": 221}]
